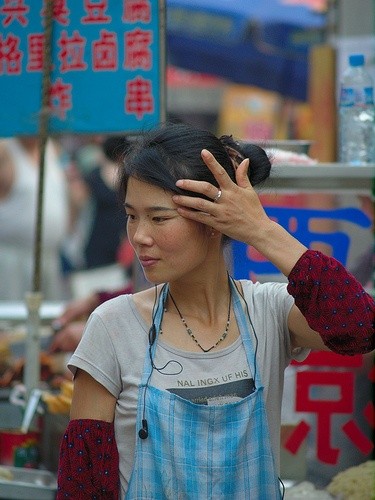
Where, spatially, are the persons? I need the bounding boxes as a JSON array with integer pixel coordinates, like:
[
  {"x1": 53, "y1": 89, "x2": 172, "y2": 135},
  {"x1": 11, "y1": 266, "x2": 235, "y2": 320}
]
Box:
[
  {"x1": 58, "y1": 121, "x2": 375, "y2": 500},
  {"x1": 83, "y1": 136, "x2": 130, "y2": 269},
  {"x1": 0, "y1": 136, "x2": 88, "y2": 300}
]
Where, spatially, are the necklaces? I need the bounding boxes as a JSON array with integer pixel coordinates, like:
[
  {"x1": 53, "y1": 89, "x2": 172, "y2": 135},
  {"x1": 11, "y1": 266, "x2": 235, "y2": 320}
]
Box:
[{"x1": 163, "y1": 271, "x2": 233, "y2": 352}]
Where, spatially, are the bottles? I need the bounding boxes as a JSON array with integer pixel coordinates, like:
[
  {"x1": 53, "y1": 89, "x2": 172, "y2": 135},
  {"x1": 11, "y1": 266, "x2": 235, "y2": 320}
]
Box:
[{"x1": 338, "y1": 55, "x2": 374, "y2": 162}]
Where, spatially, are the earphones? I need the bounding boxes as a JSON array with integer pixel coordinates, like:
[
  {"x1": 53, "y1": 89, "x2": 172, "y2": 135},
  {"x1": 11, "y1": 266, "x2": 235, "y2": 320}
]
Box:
[{"x1": 139, "y1": 420, "x2": 148, "y2": 438}]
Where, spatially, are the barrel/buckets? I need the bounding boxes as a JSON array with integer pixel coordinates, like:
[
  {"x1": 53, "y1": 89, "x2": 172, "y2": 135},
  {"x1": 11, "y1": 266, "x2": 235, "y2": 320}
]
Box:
[{"x1": 0, "y1": 427, "x2": 41, "y2": 469}]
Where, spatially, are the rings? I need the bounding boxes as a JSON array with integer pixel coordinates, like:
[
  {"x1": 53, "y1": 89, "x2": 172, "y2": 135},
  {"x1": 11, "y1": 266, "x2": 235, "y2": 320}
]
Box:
[{"x1": 213, "y1": 189, "x2": 222, "y2": 201}]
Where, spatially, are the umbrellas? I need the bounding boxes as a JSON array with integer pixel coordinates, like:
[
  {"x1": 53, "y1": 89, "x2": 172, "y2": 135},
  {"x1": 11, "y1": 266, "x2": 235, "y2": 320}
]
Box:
[{"x1": 168, "y1": 0, "x2": 327, "y2": 104}]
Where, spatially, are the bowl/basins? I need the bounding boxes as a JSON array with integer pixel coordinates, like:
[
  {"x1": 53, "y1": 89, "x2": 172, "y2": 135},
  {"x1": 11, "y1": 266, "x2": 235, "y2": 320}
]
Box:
[{"x1": 242, "y1": 140, "x2": 311, "y2": 153}]
